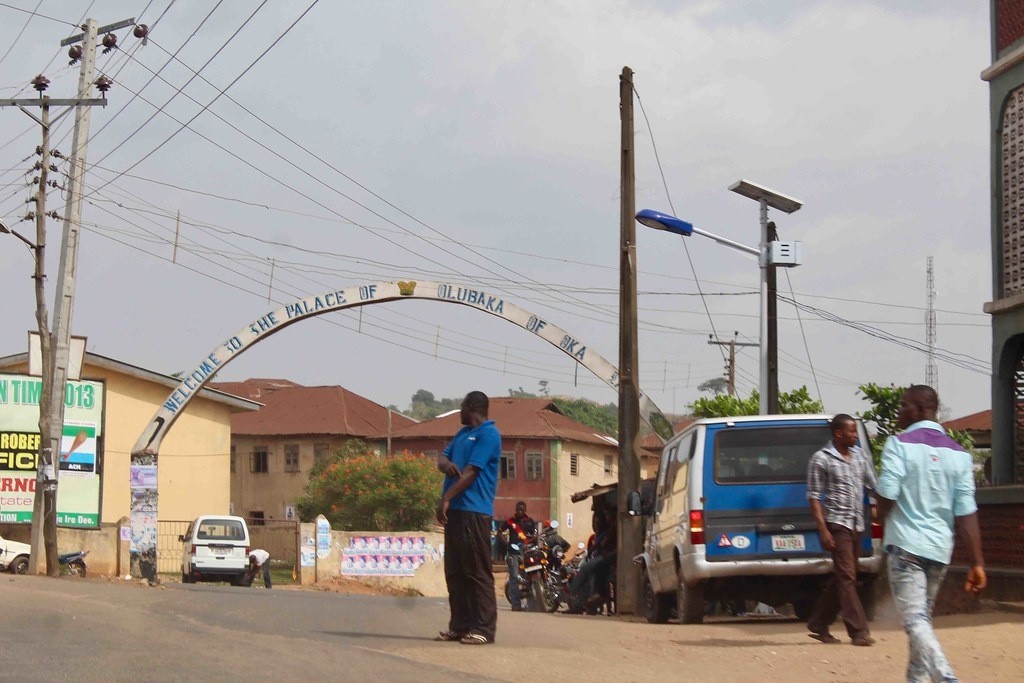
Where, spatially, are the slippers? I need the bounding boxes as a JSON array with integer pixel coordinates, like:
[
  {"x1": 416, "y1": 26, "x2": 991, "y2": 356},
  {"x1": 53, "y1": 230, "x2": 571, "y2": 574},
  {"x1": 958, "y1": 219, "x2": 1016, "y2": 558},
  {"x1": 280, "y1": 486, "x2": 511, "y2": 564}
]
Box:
[
  {"x1": 435, "y1": 630, "x2": 459, "y2": 642},
  {"x1": 460, "y1": 632, "x2": 491, "y2": 644}
]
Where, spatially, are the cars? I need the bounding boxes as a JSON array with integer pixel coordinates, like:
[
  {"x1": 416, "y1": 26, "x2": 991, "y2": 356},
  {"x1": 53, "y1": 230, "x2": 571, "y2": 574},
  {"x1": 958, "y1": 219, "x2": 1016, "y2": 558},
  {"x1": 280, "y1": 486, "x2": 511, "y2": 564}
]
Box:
[{"x1": 0, "y1": 534, "x2": 31, "y2": 576}]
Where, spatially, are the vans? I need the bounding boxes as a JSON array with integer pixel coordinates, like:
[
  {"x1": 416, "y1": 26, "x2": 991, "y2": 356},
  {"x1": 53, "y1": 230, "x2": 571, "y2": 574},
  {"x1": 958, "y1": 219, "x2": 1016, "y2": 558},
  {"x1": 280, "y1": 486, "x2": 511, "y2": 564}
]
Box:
[
  {"x1": 177, "y1": 514, "x2": 252, "y2": 587},
  {"x1": 625, "y1": 418, "x2": 884, "y2": 625}
]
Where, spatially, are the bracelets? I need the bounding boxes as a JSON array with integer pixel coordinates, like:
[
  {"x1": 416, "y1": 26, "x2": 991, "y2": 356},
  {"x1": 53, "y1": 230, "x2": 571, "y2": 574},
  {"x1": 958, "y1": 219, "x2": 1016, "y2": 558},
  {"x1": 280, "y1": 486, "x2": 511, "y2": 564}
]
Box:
[
  {"x1": 502, "y1": 540, "x2": 506, "y2": 544},
  {"x1": 872, "y1": 522, "x2": 882, "y2": 527}
]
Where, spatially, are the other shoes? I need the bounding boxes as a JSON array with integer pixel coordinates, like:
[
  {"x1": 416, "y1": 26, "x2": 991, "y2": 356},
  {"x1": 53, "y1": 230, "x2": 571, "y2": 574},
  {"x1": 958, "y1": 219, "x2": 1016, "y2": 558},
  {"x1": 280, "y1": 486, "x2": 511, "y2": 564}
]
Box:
[
  {"x1": 809, "y1": 633, "x2": 840, "y2": 643},
  {"x1": 852, "y1": 635, "x2": 875, "y2": 645},
  {"x1": 510, "y1": 606, "x2": 521, "y2": 611}
]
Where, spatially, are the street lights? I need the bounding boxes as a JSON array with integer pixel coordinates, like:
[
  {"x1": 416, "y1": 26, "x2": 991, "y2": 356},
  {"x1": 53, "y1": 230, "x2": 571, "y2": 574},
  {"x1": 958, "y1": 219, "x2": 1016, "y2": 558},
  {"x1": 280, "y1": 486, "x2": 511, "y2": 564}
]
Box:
[{"x1": 635, "y1": 209, "x2": 769, "y2": 414}]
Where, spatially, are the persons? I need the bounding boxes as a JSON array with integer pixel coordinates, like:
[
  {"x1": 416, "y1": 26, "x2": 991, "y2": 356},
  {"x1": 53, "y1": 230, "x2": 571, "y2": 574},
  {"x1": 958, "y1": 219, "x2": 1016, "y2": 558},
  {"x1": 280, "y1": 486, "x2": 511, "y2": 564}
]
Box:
[
  {"x1": 543, "y1": 522, "x2": 574, "y2": 609},
  {"x1": 587, "y1": 510, "x2": 616, "y2": 617},
  {"x1": 807, "y1": 414, "x2": 876, "y2": 647},
  {"x1": 874, "y1": 385, "x2": 986, "y2": 683},
  {"x1": 437, "y1": 391, "x2": 502, "y2": 645},
  {"x1": 497, "y1": 501, "x2": 536, "y2": 613},
  {"x1": 249, "y1": 549, "x2": 271, "y2": 589}
]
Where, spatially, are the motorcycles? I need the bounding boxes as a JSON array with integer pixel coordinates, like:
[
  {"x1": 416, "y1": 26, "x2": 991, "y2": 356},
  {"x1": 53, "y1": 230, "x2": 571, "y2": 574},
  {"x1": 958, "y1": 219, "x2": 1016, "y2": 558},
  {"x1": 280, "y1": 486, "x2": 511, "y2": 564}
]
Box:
[
  {"x1": 57, "y1": 551, "x2": 92, "y2": 580},
  {"x1": 501, "y1": 520, "x2": 587, "y2": 609}
]
